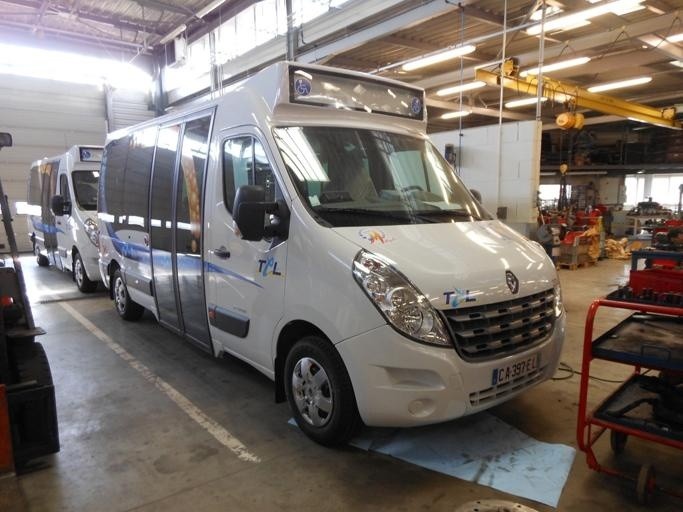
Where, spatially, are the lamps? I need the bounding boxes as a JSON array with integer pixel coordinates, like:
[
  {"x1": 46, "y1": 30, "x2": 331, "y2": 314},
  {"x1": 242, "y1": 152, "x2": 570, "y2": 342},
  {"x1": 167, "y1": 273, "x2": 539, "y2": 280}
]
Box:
[{"x1": 399, "y1": 1, "x2": 683, "y2": 123}]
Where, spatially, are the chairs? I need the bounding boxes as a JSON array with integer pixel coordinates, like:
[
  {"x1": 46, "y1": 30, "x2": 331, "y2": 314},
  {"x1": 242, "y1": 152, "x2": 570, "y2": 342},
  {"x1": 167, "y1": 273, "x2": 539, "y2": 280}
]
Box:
[{"x1": 334, "y1": 138, "x2": 380, "y2": 202}]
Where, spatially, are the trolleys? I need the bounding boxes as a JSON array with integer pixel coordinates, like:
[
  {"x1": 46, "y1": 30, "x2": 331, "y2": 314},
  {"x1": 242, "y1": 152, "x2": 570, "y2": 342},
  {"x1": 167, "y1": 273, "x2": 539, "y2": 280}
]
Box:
[{"x1": 568, "y1": 248, "x2": 683, "y2": 510}]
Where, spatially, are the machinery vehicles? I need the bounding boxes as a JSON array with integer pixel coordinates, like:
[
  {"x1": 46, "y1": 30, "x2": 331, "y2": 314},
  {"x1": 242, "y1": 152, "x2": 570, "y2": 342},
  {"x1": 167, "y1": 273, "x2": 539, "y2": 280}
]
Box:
[{"x1": 0, "y1": 131, "x2": 64, "y2": 466}]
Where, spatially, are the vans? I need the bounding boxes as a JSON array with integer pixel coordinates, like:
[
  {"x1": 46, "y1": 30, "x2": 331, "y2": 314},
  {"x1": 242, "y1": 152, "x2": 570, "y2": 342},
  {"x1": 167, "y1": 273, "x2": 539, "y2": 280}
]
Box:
[
  {"x1": 22, "y1": 140, "x2": 101, "y2": 293},
  {"x1": 89, "y1": 58, "x2": 569, "y2": 446}
]
